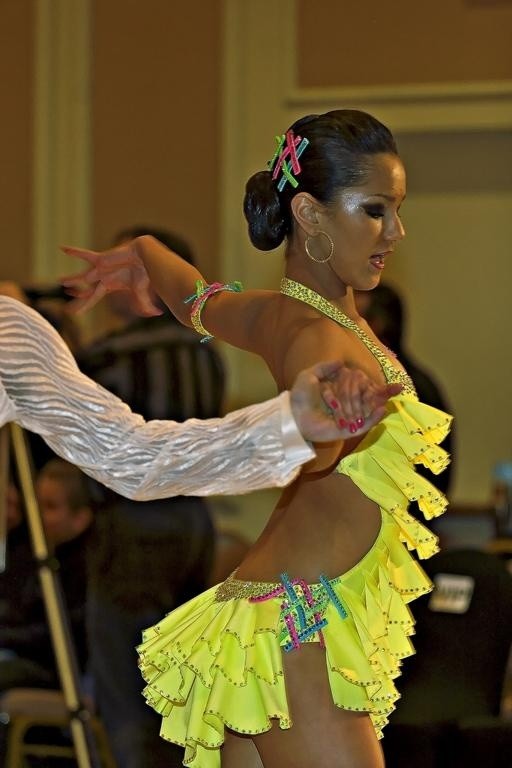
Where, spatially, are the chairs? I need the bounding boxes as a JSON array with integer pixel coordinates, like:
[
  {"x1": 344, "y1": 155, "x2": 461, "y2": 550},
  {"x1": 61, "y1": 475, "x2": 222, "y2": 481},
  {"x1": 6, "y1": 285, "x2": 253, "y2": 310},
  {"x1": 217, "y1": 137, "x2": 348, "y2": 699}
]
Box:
[
  {"x1": 1, "y1": 482, "x2": 217, "y2": 768},
  {"x1": 70, "y1": 320, "x2": 231, "y2": 662}
]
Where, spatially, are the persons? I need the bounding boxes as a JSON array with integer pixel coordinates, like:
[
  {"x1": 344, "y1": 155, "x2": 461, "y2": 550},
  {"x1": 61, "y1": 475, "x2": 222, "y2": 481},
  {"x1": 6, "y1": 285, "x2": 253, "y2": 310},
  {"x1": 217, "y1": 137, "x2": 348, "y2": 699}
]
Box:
[
  {"x1": 57, "y1": 110, "x2": 455, "y2": 768},
  {"x1": 354, "y1": 279, "x2": 456, "y2": 740},
  {"x1": 0, "y1": 228, "x2": 225, "y2": 768},
  {"x1": 0, "y1": 298, "x2": 402, "y2": 504}
]
